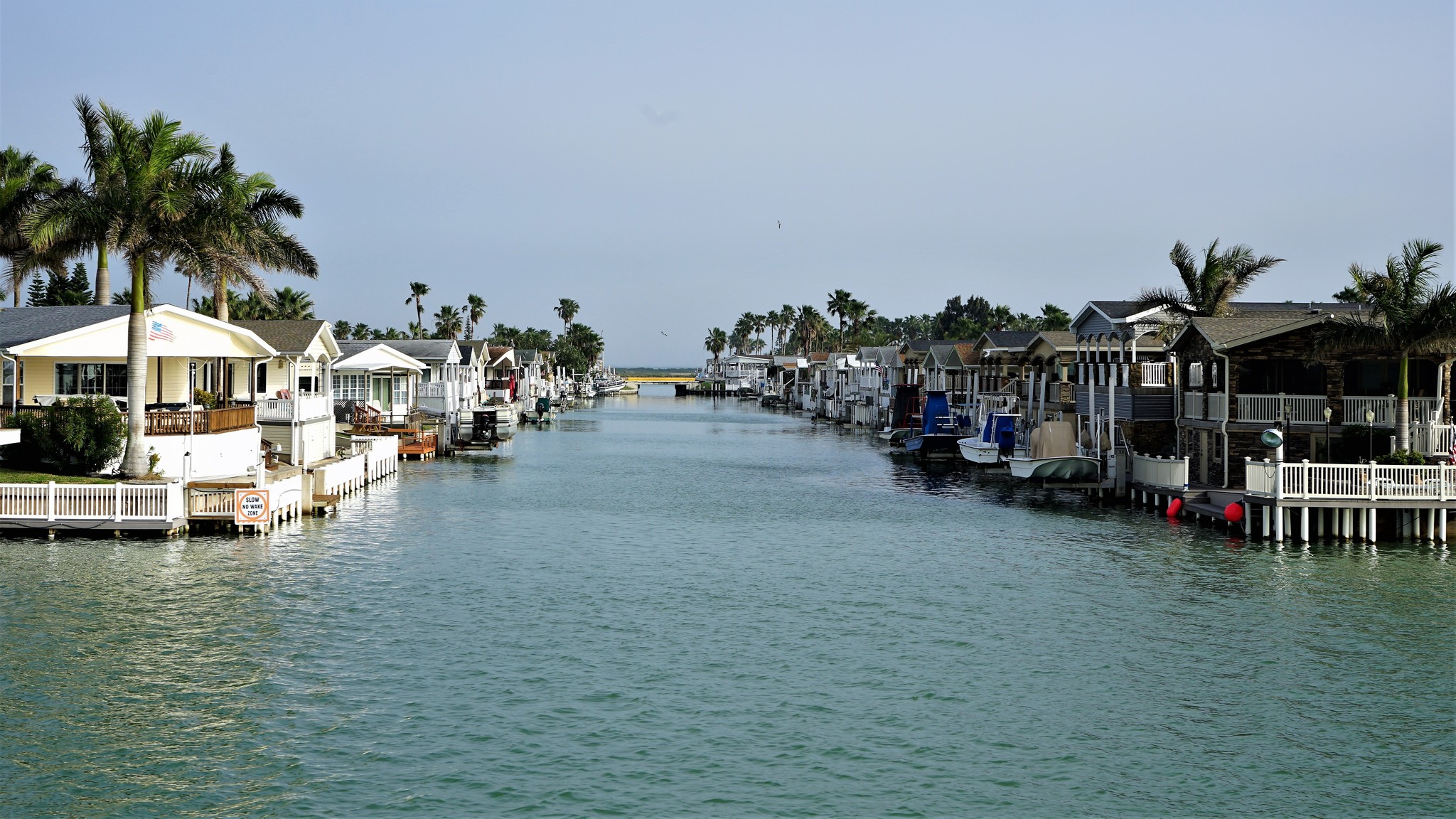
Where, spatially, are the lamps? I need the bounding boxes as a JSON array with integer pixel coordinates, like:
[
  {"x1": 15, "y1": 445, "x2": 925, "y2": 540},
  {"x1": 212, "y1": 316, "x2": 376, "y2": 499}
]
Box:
[
  {"x1": 264, "y1": 443, "x2": 282, "y2": 456},
  {"x1": 1324, "y1": 407, "x2": 1332, "y2": 419},
  {"x1": 1260, "y1": 420, "x2": 1287, "y2": 448},
  {"x1": 231, "y1": 401, "x2": 236, "y2": 408},
  {"x1": 17, "y1": 398, "x2": 23, "y2": 405},
  {"x1": 1366, "y1": 410, "x2": 1375, "y2": 422},
  {"x1": 205, "y1": 404, "x2": 210, "y2": 411},
  {"x1": 1285, "y1": 403, "x2": 1292, "y2": 414},
  {"x1": 185, "y1": 452, "x2": 190, "y2": 456}
]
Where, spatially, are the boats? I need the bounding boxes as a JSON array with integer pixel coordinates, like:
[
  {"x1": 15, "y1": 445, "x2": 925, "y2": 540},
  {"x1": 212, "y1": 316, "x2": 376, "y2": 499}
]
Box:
[
  {"x1": 573, "y1": 365, "x2": 626, "y2": 408},
  {"x1": 957, "y1": 396, "x2": 1029, "y2": 463},
  {"x1": 1005, "y1": 420, "x2": 1104, "y2": 479},
  {"x1": 457, "y1": 405, "x2": 520, "y2": 442},
  {"x1": 757, "y1": 374, "x2": 781, "y2": 402},
  {"x1": 737, "y1": 383, "x2": 755, "y2": 397},
  {"x1": 525, "y1": 396, "x2": 559, "y2": 424},
  {"x1": 551, "y1": 391, "x2": 576, "y2": 408},
  {"x1": 876, "y1": 384, "x2": 974, "y2": 458},
  {"x1": 615, "y1": 388, "x2": 638, "y2": 394}
]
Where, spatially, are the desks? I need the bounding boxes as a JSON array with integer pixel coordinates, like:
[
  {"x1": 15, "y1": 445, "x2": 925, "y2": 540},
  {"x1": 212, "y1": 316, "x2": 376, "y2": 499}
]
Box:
[
  {"x1": 350, "y1": 439, "x2": 373, "y2": 460},
  {"x1": 344, "y1": 423, "x2": 381, "y2": 436}
]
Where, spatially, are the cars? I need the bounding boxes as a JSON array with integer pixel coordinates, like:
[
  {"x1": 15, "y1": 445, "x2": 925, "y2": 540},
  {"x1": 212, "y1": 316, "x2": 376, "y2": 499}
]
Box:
[{"x1": 695, "y1": 372, "x2": 723, "y2": 378}]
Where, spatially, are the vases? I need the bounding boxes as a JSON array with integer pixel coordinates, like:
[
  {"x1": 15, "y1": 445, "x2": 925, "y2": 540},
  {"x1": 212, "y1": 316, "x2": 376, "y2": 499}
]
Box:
[{"x1": 1351, "y1": 473, "x2": 1367, "y2": 486}]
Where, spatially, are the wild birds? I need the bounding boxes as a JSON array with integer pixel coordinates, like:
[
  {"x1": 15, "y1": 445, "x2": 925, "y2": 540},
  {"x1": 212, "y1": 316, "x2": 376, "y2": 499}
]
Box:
[
  {"x1": 777, "y1": 221, "x2": 781, "y2": 229},
  {"x1": 661, "y1": 332, "x2": 668, "y2": 336}
]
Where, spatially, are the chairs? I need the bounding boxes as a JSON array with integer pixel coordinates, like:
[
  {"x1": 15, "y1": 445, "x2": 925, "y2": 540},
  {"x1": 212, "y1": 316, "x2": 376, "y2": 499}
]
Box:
[
  {"x1": 373, "y1": 400, "x2": 394, "y2": 422},
  {"x1": 280, "y1": 389, "x2": 300, "y2": 415}
]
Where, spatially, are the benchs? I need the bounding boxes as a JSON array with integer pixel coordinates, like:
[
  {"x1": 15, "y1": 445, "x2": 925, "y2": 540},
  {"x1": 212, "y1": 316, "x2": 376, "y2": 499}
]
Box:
[{"x1": 343, "y1": 429, "x2": 385, "y2": 432}]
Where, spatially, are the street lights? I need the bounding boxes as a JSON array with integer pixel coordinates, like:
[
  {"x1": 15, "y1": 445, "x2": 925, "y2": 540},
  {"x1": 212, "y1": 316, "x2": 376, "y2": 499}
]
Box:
[
  {"x1": 1324, "y1": 404, "x2": 1331, "y2": 491},
  {"x1": 1284, "y1": 402, "x2": 1292, "y2": 462},
  {"x1": 1365, "y1": 410, "x2": 1374, "y2": 461}
]
